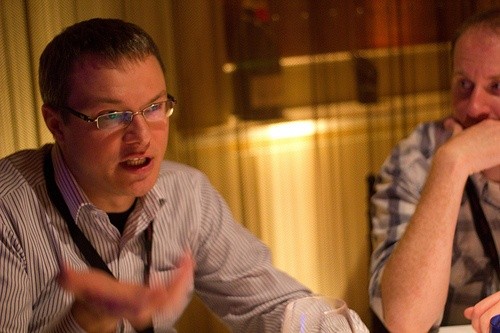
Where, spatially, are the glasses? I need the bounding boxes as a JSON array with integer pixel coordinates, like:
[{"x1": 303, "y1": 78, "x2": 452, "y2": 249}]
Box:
[{"x1": 50, "y1": 94, "x2": 176, "y2": 131}]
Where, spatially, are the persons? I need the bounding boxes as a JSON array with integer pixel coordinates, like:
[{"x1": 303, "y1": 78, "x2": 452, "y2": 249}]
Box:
[
  {"x1": 368, "y1": 8, "x2": 500, "y2": 333},
  {"x1": 0, "y1": 16, "x2": 368, "y2": 333}
]
